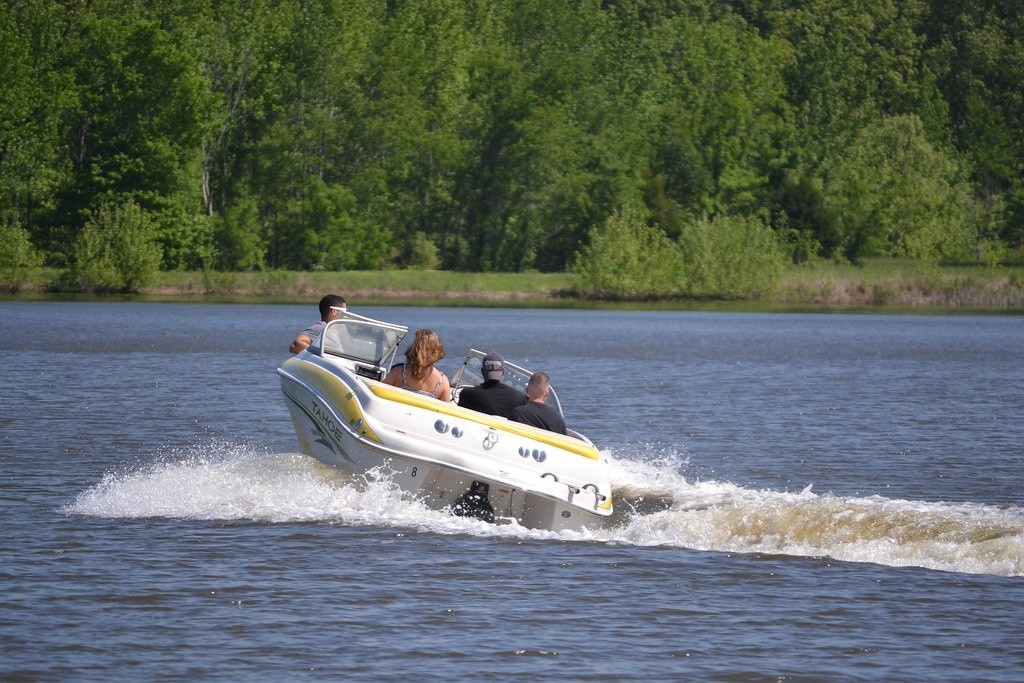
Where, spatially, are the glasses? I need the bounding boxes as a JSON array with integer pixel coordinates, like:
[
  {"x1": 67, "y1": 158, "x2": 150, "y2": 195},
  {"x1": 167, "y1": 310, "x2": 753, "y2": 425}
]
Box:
[{"x1": 330, "y1": 306, "x2": 348, "y2": 312}]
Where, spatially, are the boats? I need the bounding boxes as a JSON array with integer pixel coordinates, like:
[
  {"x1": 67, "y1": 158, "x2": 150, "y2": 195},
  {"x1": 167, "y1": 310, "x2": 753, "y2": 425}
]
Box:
[{"x1": 277, "y1": 308, "x2": 614, "y2": 534}]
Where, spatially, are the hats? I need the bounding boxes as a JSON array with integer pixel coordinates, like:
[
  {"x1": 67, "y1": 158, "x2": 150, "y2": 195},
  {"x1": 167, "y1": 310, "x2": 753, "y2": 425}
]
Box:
[{"x1": 482, "y1": 352, "x2": 504, "y2": 382}]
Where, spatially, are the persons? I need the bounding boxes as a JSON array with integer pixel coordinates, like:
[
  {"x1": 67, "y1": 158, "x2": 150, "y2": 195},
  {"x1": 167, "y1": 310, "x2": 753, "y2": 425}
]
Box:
[
  {"x1": 382, "y1": 329, "x2": 450, "y2": 406},
  {"x1": 511, "y1": 371, "x2": 567, "y2": 435},
  {"x1": 458, "y1": 353, "x2": 532, "y2": 419},
  {"x1": 288, "y1": 294, "x2": 350, "y2": 358}
]
